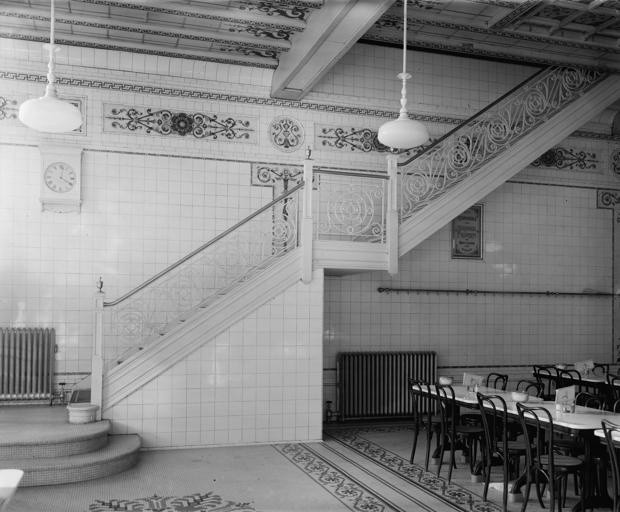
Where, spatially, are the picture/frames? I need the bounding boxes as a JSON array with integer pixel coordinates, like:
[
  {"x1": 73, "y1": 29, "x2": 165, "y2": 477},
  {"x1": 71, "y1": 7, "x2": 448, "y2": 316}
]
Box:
[{"x1": 449, "y1": 203, "x2": 483, "y2": 261}]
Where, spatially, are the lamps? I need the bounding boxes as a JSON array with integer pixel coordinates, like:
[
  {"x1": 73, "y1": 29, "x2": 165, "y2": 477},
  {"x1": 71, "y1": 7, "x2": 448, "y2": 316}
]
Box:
[
  {"x1": 17, "y1": 0, "x2": 84, "y2": 134},
  {"x1": 377, "y1": 1, "x2": 430, "y2": 151}
]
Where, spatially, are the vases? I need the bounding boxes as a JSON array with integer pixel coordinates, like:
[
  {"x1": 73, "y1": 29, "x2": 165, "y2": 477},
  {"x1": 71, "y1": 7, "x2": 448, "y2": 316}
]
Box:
[{"x1": 65, "y1": 401, "x2": 100, "y2": 423}]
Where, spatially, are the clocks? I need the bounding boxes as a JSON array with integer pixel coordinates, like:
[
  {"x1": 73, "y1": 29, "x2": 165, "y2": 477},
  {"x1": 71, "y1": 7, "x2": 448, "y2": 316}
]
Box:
[{"x1": 43, "y1": 159, "x2": 77, "y2": 194}]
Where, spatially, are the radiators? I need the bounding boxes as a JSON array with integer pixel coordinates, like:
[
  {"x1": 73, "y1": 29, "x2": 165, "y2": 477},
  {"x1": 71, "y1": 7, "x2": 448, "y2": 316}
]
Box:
[
  {"x1": 0, "y1": 327, "x2": 56, "y2": 405},
  {"x1": 335, "y1": 350, "x2": 438, "y2": 425}
]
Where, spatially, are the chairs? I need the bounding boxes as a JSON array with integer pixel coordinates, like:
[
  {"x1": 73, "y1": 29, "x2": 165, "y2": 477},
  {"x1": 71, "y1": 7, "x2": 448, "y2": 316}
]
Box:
[{"x1": 408, "y1": 363, "x2": 620, "y2": 512}]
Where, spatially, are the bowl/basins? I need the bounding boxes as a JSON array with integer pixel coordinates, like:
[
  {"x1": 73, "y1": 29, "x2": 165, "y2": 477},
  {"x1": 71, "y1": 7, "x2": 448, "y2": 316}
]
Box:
[
  {"x1": 438, "y1": 376, "x2": 454, "y2": 386},
  {"x1": 512, "y1": 390, "x2": 530, "y2": 403},
  {"x1": 66, "y1": 403, "x2": 99, "y2": 424}
]
